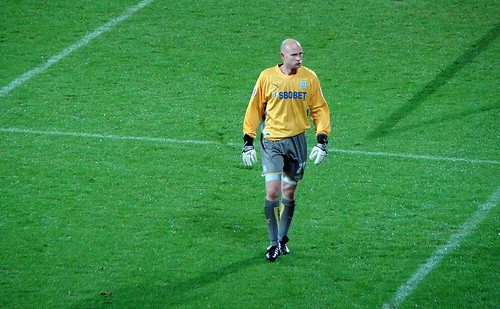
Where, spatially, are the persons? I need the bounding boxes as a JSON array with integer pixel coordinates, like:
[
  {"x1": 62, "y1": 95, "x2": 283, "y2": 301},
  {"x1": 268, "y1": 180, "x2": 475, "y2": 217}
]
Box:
[{"x1": 242, "y1": 38, "x2": 331, "y2": 262}]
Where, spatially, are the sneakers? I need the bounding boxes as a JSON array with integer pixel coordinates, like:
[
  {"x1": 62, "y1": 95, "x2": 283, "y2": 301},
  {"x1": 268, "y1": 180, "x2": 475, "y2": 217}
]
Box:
[
  {"x1": 278, "y1": 235, "x2": 290, "y2": 254},
  {"x1": 265, "y1": 246, "x2": 280, "y2": 260}
]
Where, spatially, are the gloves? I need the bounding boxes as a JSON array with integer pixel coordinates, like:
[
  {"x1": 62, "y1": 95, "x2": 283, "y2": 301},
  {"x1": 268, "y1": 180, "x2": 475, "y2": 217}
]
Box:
[
  {"x1": 242, "y1": 144, "x2": 257, "y2": 168},
  {"x1": 309, "y1": 143, "x2": 328, "y2": 165}
]
